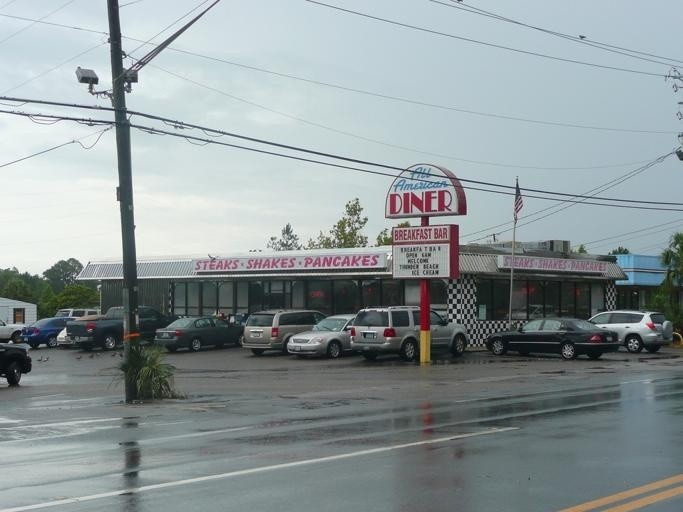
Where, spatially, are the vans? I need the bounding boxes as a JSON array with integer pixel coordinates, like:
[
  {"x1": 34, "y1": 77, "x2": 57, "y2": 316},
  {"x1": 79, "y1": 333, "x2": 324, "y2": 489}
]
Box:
[
  {"x1": 241, "y1": 309, "x2": 328, "y2": 355},
  {"x1": 54, "y1": 308, "x2": 100, "y2": 320}
]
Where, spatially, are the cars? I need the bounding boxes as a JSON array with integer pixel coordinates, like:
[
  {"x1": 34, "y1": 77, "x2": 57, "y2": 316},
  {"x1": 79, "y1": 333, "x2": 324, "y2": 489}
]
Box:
[
  {"x1": 20, "y1": 318, "x2": 75, "y2": 347},
  {"x1": 56, "y1": 327, "x2": 97, "y2": 348},
  {"x1": 0, "y1": 343, "x2": 31, "y2": 384},
  {"x1": 485, "y1": 317, "x2": 621, "y2": 359},
  {"x1": 286, "y1": 314, "x2": 357, "y2": 358},
  {"x1": 0, "y1": 319, "x2": 30, "y2": 342},
  {"x1": 155, "y1": 316, "x2": 244, "y2": 352}
]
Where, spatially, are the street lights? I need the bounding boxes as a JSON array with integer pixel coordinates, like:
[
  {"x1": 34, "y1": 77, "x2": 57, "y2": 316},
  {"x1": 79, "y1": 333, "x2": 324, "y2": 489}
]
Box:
[{"x1": 77, "y1": 64, "x2": 142, "y2": 405}]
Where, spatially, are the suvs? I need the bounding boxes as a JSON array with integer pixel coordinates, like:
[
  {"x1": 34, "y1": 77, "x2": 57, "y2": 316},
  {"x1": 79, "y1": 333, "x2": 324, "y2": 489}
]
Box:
[
  {"x1": 350, "y1": 305, "x2": 469, "y2": 362},
  {"x1": 588, "y1": 310, "x2": 673, "y2": 353}
]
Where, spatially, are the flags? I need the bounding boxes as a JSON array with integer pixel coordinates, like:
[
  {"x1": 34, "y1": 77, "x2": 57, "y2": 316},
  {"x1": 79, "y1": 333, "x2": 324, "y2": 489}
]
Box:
[{"x1": 514, "y1": 179, "x2": 523, "y2": 224}]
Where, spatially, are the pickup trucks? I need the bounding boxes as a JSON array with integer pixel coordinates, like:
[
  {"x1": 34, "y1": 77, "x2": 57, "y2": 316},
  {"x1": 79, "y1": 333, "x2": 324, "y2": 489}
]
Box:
[{"x1": 65, "y1": 306, "x2": 180, "y2": 351}]
